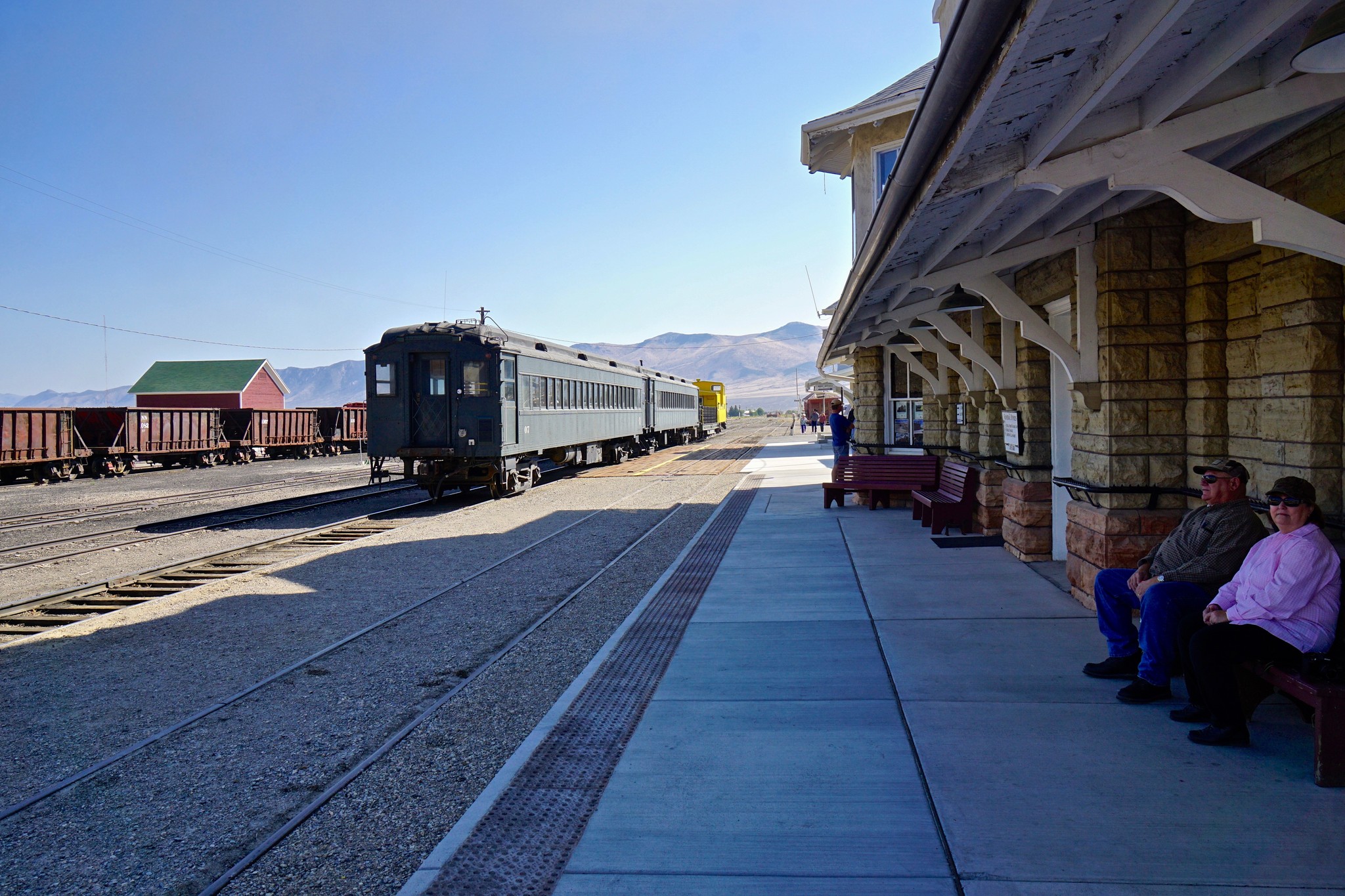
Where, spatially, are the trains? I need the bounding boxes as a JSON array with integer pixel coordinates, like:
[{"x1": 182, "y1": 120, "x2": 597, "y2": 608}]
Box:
[
  {"x1": 0, "y1": 402, "x2": 367, "y2": 484},
  {"x1": 363, "y1": 306, "x2": 728, "y2": 502}
]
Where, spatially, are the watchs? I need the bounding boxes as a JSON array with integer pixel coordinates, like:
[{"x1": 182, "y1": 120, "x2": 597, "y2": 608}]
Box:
[{"x1": 1157, "y1": 574, "x2": 1165, "y2": 583}]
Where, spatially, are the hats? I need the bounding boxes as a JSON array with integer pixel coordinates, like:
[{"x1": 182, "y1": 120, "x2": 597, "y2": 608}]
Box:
[
  {"x1": 1265, "y1": 476, "x2": 1316, "y2": 502},
  {"x1": 1193, "y1": 459, "x2": 1250, "y2": 479},
  {"x1": 831, "y1": 399, "x2": 844, "y2": 406}
]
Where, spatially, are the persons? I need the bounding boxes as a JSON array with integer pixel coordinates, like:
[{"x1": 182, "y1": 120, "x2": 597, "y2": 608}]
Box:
[
  {"x1": 1170, "y1": 477, "x2": 1344, "y2": 750},
  {"x1": 829, "y1": 399, "x2": 855, "y2": 494},
  {"x1": 1081, "y1": 457, "x2": 1270, "y2": 705},
  {"x1": 800, "y1": 413, "x2": 810, "y2": 434},
  {"x1": 819, "y1": 411, "x2": 827, "y2": 432},
  {"x1": 810, "y1": 409, "x2": 820, "y2": 433},
  {"x1": 791, "y1": 413, "x2": 795, "y2": 420}
]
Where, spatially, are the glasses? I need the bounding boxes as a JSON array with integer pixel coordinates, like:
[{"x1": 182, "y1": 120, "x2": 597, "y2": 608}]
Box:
[
  {"x1": 1201, "y1": 475, "x2": 1233, "y2": 484},
  {"x1": 1267, "y1": 496, "x2": 1311, "y2": 507}
]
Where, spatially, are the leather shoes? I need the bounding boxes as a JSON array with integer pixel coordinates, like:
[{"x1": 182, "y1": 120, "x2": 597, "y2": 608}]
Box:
[
  {"x1": 1117, "y1": 678, "x2": 1172, "y2": 702},
  {"x1": 1187, "y1": 725, "x2": 1249, "y2": 745},
  {"x1": 1082, "y1": 648, "x2": 1144, "y2": 679},
  {"x1": 1169, "y1": 705, "x2": 1217, "y2": 723}
]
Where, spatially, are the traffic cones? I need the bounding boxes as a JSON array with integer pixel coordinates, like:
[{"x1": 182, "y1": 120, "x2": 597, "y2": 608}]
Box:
[{"x1": 783, "y1": 419, "x2": 784, "y2": 421}]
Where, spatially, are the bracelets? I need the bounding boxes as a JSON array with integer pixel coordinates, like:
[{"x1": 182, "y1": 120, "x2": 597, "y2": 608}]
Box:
[{"x1": 1225, "y1": 612, "x2": 1229, "y2": 621}]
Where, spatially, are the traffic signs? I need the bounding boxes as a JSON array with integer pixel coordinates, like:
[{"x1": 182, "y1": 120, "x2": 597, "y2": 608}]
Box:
[
  {"x1": 794, "y1": 399, "x2": 808, "y2": 402},
  {"x1": 815, "y1": 384, "x2": 836, "y2": 391}
]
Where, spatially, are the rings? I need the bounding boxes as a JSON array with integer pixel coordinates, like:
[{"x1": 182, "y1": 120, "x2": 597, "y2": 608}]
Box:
[
  {"x1": 1138, "y1": 594, "x2": 1140, "y2": 596},
  {"x1": 1210, "y1": 621, "x2": 1214, "y2": 626}
]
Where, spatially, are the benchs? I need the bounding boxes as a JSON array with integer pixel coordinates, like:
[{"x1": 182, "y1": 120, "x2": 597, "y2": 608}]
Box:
[
  {"x1": 1262, "y1": 645, "x2": 1345, "y2": 788},
  {"x1": 822, "y1": 455, "x2": 940, "y2": 519},
  {"x1": 911, "y1": 460, "x2": 975, "y2": 535}
]
